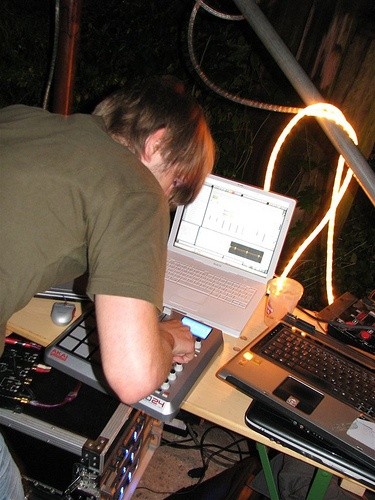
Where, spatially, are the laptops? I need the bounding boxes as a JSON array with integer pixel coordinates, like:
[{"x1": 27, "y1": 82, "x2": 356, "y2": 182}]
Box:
[
  {"x1": 244, "y1": 399, "x2": 375, "y2": 488},
  {"x1": 215, "y1": 312, "x2": 375, "y2": 473},
  {"x1": 163, "y1": 174, "x2": 296, "y2": 339}
]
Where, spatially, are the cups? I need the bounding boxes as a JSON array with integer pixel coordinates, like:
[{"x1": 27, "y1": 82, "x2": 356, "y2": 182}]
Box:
[{"x1": 264, "y1": 277, "x2": 304, "y2": 327}]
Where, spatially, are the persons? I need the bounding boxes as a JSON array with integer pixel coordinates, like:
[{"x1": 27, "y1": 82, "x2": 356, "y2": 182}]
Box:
[{"x1": 0, "y1": 83, "x2": 215, "y2": 500}]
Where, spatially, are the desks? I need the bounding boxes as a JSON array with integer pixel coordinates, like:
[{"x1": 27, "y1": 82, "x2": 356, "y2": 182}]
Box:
[{"x1": 5, "y1": 296, "x2": 375, "y2": 500}]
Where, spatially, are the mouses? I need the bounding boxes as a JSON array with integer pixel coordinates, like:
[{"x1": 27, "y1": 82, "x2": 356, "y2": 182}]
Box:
[{"x1": 50, "y1": 302, "x2": 76, "y2": 327}]
